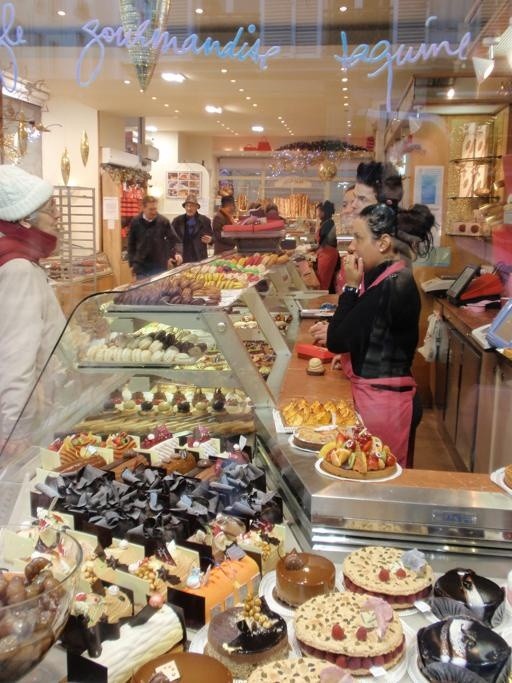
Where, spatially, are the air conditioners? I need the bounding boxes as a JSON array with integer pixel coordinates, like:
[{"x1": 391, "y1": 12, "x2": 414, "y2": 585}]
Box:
[{"x1": 102, "y1": 147, "x2": 140, "y2": 169}]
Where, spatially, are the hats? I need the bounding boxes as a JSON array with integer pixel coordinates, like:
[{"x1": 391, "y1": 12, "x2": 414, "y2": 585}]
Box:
[
  {"x1": 182, "y1": 195, "x2": 200, "y2": 209},
  {"x1": 0, "y1": 163, "x2": 54, "y2": 222}
]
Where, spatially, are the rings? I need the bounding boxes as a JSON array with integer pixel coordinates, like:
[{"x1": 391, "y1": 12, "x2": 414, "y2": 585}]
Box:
[{"x1": 344, "y1": 260, "x2": 350, "y2": 265}]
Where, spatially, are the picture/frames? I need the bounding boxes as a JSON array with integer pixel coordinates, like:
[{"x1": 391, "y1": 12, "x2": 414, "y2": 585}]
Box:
[{"x1": 485, "y1": 297, "x2": 512, "y2": 348}]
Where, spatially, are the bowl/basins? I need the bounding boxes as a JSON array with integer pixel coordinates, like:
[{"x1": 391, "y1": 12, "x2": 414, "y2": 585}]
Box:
[{"x1": 2, "y1": 523, "x2": 82, "y2": 682}]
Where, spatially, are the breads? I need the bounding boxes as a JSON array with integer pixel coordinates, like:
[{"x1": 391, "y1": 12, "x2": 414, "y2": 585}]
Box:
[
  {"x1": 234, "y1": 311, "x2": 293, "y2": 332},
  {"x1": 213, "y1": 252, "x2": 291, "y2": 268},
  {"x1": 180, "y1": 264, "x2": 259, "y2": 290},
  {"x1": 502, "y1": 466, "x2": 512, "y2": 489},
  {"x1": 174, "y1": 339, "x2": 277, "y2": 381},
  {"x1": 84, "y1": 329, "x2": 206, "y2": 366},
  {"x1": 114, "y1": 276, "x2": 224, "y2": 309}
]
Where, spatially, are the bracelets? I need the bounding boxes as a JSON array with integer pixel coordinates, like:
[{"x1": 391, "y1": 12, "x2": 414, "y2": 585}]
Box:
[{"x1": 344, "y1": 285, "x2": 361, "y2": 295}]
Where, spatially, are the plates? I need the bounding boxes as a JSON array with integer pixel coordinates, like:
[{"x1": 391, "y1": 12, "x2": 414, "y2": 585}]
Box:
[
  {"x1": 256, "y1": 569, "x2": 331, "y2": 616},
  {"x1": 188, "y1": 621, "x2": 300, "y2": 661},
  {"x1": 424, "y1": 583, "x2": 510, "y2": 634},
  {"x1": 334, "y1": 565, "x2": 433, "y2": 616},
  {"x1": 491, "y1": 468, "x2": 512, "y2": 498},
  {"x1": 408, "y1": 653, "x2": 435, "y2": 683},
  {"x1": 316, "y1": 451, "x2": 403, "y2": 482},
  {"x1": 285, "y1": 431, "x2": 335, "y2": 452}
]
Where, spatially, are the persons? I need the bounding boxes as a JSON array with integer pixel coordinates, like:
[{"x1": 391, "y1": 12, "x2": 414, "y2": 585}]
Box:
[
  {"x1": 236, "y1": 202, "x2": 264, "y2": 225},
  {"x1": 0, "y1": 162, "x2": 78, "y2": 464},
  {"x1": 335, "y1": 185, "x2": 365, "y2": 298},
  {"x1": 166, "y1": 194, "x2": 216, "y2": 270},
  {"x1": 212, "y1": 195, "x2": 237, "y2": 257},
  {"x1": 323, "y1": 201, "x2": 438, "y2": 470},
  {"x1": 308, "y1": 159, "x2": 405, "y2": 350},
  {"x1": 308, "y1": 200, "x2": 341, "y2": 294},
  {"x1": 126, "y1": 195, "x2": 184, "y2": 281},
  {"x1": 265, "y1": 202, "x2": 287, "y2": 224}
]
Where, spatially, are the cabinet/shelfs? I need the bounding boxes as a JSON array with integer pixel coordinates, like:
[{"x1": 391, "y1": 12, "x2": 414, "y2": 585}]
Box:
[
  {"x1": 443, "y1": 157, "x2": 494, "y2": 239},
  {"x1": 446, "y1": 305, "x2": 512, "y2": 471},
  {"x1": 51, "y1": 184, "x2": 96, "y2": 305},
  {"x1": 0, "y1": 248, "x2": 307, "y2": 465},
  {"x1": 101, "y1": 168, "x2": 147, "y2": 286},
  {"x1": 426, "y1": 295, "x2": 450, "y2": 435},
  {"x1": 1, "y1": 407, "x2": 511, "y2": 683}
]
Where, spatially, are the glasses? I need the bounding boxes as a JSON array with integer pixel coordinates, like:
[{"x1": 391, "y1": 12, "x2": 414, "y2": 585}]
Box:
[{"x1": 37, "y1": 203, "x2": 55, "y2": 217}]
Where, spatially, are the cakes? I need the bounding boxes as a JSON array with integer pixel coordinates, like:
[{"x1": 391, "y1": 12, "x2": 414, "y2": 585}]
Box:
[
  {"x1": 235, "y1": 528, "x2": 286, "y2": 573},
  {"x1": 31, "y1": 424, "x2": 283, "y2": 551},
  {"x1": 292, "y1": 591, "x2": 405, "y2": 677},
  {"x1": 98, "y1": 537, "x2": 146, "y2": 573},
  {"x1": 103, "y1": 386, "x2": 250, "y2": 414},
  {"x1": 341, "y1": 547, "x2": 433, "y2": 610},
  {"x1": 415, "y1": 615, "x2": 512, "y2": 683},
  {"x1": 132, "y1": 653, "x2": 232, "y2": 682},
  {"x1": 137, "y1": 541, "x2": 201, "y2": 601},
  {"x1": 168, "y1": 553, "x2": 264, "y2": 628},
  {"x1": 282, "y1": 397, "x2": 361, "y2": 429},
  {"x1": 422, "y1": 568, "x2": 505, "y2": 628},
  {"x1": 293, "y1": 426, "x2": 367, "y2": 451},
  {"x1": 247, "y1": 658, "x2": 357, "y2": 683},
  {"x1": 203, "y1": 593, "x2": 290, "y2": 681},
  {"x1": 60, "y1": 528, "x2": 103, "y2": 560},
  {"x1": 276, "y1": 551, "x2": 336, "y2": 610},
  {"x1": 319, "y1": 429, "x2": 397, "y2": 480}
]
